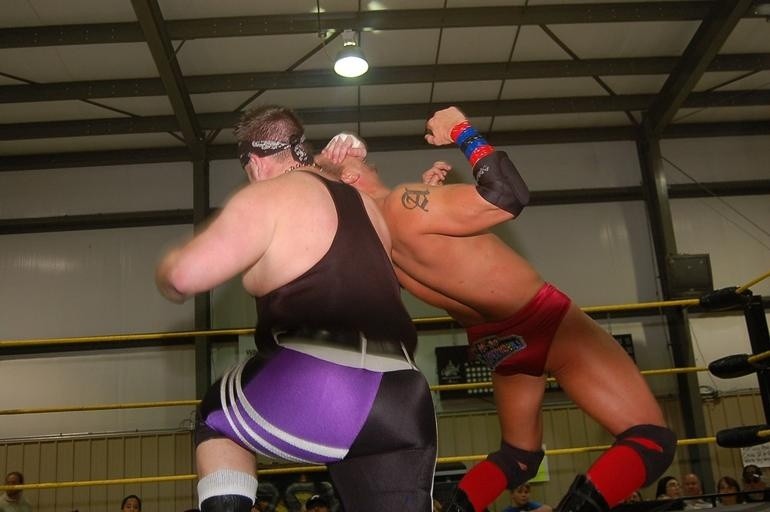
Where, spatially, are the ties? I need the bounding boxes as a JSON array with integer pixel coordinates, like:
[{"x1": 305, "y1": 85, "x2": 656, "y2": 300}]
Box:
[{"x1": 664, "y1": 252, "x2": 713, "y2": 299}]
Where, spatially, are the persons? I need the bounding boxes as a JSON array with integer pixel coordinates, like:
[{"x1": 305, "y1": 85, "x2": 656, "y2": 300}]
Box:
[
  {"x1": 151, "y1": 102, "x2": 443, "y2": 511},
  {"x1": 678, "y1": 473, "x2": 714, "y2": 509},
  {"x1": 716, "y1": 476, "x2": 747, "y2": 504},
  {"x1": 622, "y1": 490, "x2": 644, "y2": 503},
  {"x1": 251, "y1": 494, "x2": 273, "y2": 511},
  {"x1": 737, "y1": 464, "x2": 770, "y2": 502},
  {"x1": 305, "y1": 493, "x2": 331, "y2": 511},
  {"x1": 0, "y1": 471, "x2": 33, "y2": 512},
  {"x1": 120, "y1": 494, "x2": 142, "y2": 512},
  {"x1": 654, "y1": 475, "x2": 681, "y2": 498},
  {"x1": 498, "y1": 480, "x2": 553, "y2": 511},
  {"x1": 308, "y1": 104, "x2": 681, "y2": 511}
]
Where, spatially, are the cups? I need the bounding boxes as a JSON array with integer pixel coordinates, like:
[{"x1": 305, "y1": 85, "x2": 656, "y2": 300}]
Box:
[{"x1": 744, "y1": 477, "x2": 759, "y2": 484}]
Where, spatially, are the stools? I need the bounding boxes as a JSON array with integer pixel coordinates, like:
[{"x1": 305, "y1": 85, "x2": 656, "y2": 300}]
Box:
[{"x1": 277, "y1": 328, "x2": 415, "y2": 363}]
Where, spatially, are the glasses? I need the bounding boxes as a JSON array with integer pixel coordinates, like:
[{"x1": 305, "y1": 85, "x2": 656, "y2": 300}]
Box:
[{"x1": 334, "y1": 29, "x2": 369, "y2": 78}]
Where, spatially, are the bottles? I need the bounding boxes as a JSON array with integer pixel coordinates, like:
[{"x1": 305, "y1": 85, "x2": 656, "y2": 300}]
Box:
[
  {"x1": 743, "y1": 465, "x2": 762, "y2": 477},
  {"x1": 306, "y1": 493, "x2": 327, "y2": 509}
]
